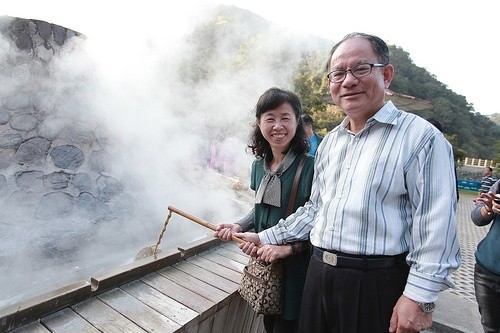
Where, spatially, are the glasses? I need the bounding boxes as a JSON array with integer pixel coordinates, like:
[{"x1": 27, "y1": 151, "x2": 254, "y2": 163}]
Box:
[{"x1": 326, "y1": 63, "x2": 386, "y2": 83}]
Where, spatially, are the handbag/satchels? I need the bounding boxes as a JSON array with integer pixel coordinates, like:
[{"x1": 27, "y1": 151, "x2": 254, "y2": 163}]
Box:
[{"x1": 238, "y1": 256, "x2": 285, "y2": 316}]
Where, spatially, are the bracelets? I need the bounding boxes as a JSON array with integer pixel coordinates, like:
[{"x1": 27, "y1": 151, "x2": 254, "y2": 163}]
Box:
[{"x1": 484, "y1": 204, "x2": 492, "y2": 215}]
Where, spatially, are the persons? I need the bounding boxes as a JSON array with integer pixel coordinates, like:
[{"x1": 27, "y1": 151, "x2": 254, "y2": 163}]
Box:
[
  {"x1": 211, "y1": 133, "x2": 240, "y2": 177},
  {"x1": 214, "y1": 83, "x2": 315, "y2": 333},
  {"x1": 298, "y1": 114, "x2": 323, "y2": 156},
  {"x1": 234, "y1": 32, "x2": 461, "y2": 333},
  {"x1": 478, "y1": 167, "x2": 497, "y2": 197},
  {"x1": 427, "y1": 119, "x2": 459, "y2": 202},
  {"x1": 471, "y1": 177, "x2": 500, "y2": 333}
]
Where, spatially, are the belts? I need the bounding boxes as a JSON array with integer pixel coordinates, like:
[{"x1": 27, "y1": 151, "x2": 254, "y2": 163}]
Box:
[{"x1": 312, "y1": 247, "x2": 409, "y2": 270}]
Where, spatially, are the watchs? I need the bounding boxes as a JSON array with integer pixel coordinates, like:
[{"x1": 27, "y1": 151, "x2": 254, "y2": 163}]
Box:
[{"x1": 415, "y1": 301, "x2": 435, "y2": 313}]
